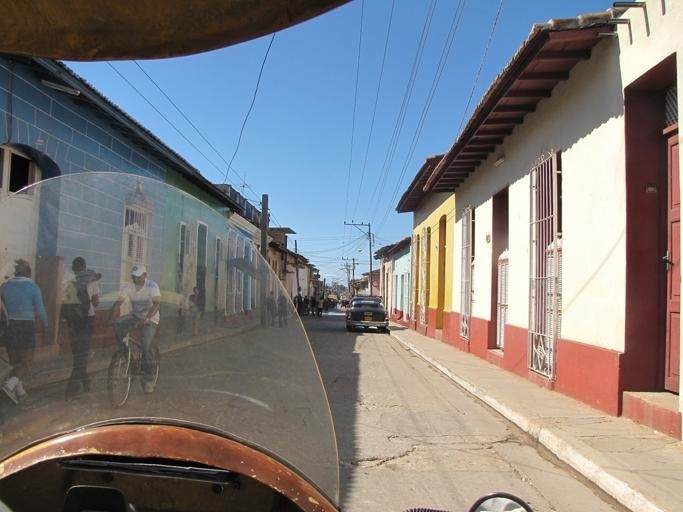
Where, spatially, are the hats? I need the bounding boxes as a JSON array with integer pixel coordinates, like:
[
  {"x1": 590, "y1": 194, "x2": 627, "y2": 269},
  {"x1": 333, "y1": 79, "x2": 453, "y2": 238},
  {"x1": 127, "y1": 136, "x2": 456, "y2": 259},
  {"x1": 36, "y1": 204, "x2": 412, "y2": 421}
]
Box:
[{"x1": 129, "y1": 265, "x2": 146, "y2": 277}]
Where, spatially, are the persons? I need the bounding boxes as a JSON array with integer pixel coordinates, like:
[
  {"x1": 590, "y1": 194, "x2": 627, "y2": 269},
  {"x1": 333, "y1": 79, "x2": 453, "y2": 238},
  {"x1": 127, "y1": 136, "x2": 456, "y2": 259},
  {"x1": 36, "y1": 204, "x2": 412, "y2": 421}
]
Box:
[
  {"x1": 0, "y1": 258, "x2": 49, "y2": 407},
  {"x1": 268, "y1": 291, "x2": 276, "y2": 327},
  {"x1": 188, "y1": 286, "x2": 203, "y2": 336},
  {"x1": 56, "y1": 256, "x2": 99, "y2": 394},
  {"x1": 277, "y1": 290, "x2": 288, "y2": 328},
  {"x1": 299, "y1": 293, "x2": 331, "y2": 317},
  {"x1": 109, "y1": 264, "x2": 160, "y2": 382}
]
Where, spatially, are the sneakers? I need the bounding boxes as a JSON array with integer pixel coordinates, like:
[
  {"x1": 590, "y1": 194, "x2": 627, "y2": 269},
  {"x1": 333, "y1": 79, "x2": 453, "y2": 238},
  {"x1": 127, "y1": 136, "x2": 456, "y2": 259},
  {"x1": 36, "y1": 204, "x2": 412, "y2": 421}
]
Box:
[
  {"x1": 2, "y1": 385, "x2": 17, "y2": 405},
  {"x1": 143, "y1": 383, "x2": 153, "y2": 393},
  {"x1": 17, "y1": 392, "x2": 28, "y2": 402}
]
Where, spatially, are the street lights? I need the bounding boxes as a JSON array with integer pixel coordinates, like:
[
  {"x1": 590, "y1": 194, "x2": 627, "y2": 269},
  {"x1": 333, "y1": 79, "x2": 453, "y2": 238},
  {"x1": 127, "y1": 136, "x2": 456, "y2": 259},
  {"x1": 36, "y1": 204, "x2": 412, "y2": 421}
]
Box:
[{"x1": 357, "y1": 248, "x2": 372, "y2": 296}]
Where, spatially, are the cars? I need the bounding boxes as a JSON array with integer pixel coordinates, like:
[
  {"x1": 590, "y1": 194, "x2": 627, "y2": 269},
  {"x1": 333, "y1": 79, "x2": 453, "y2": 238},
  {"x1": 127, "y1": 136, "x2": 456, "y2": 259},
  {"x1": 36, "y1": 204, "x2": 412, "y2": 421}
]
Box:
[{"x1": 346, "y1": 295, "x2": 389, "y2": 331}]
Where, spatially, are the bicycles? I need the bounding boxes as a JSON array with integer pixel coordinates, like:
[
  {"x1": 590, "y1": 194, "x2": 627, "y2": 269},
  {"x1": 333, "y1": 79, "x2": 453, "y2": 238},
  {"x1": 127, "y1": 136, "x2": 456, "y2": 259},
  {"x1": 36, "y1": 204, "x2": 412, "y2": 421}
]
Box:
[{"x1": 108, "y1": 321, "x2": 159, "y2": 408}]
